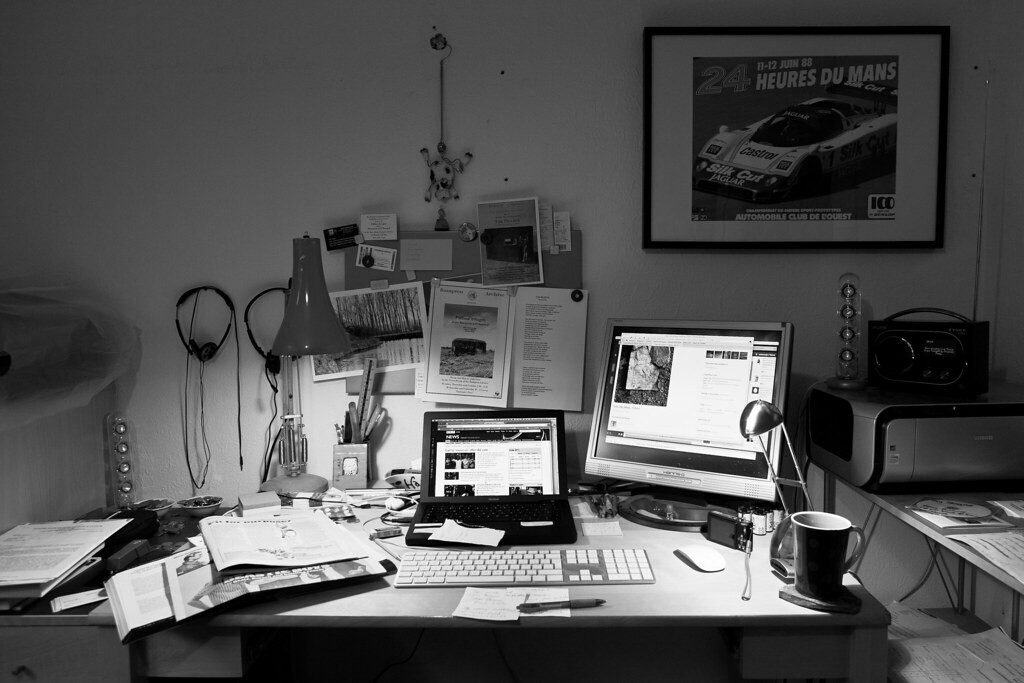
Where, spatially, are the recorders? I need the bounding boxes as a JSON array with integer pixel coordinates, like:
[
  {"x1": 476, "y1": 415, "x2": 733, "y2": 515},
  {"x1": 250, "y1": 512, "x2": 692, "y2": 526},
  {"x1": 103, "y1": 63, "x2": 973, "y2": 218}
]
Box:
[{"x1": 867, "y1": 320, "x2": 989, "y2": 402}]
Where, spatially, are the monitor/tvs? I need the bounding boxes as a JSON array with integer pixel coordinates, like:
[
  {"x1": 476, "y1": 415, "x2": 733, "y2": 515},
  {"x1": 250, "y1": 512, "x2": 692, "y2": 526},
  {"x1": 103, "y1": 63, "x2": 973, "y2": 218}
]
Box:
[{"x1": 585, "y1": 318, "x2": 794, "y2": 533}]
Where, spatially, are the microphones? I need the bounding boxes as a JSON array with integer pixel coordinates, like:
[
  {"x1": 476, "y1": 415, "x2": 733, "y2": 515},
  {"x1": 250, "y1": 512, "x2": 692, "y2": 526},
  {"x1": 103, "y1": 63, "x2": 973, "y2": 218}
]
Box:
[{"x1": 265, "y1": 367, "x2": 278, "y2": 393}]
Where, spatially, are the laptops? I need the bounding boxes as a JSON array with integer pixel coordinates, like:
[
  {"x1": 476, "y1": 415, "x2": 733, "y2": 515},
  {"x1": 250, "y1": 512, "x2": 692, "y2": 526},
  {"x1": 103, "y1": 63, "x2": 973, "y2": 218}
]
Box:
[{"x1": 405, "y1": 408, "x2": 577, "y2": 547}]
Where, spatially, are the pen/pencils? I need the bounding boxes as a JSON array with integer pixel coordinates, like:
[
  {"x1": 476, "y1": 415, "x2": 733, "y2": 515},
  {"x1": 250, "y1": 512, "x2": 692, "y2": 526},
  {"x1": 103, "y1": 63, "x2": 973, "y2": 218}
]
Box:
[
  {"x1": 332, "y1": 400, "x2": 386, "y2": 444},
  {"x1": 516, "y1": 598, "x2": 606, "y2": 613},
  {"x1": 587, "y1": 493, "x2": 620, "y2": 518}
]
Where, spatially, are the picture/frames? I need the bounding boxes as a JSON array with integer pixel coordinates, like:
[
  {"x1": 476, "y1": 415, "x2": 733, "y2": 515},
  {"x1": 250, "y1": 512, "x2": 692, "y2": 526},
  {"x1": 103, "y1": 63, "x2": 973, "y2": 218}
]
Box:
[{"x1": 642, "y1": 24, "x2": 953, "y2": 250}]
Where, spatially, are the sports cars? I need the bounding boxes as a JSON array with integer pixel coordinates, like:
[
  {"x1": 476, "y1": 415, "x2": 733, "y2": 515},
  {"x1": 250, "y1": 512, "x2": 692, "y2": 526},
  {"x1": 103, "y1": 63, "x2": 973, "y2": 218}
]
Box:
[{"x1": 693, "y1": 93, "x2": 899, "y2": 202}]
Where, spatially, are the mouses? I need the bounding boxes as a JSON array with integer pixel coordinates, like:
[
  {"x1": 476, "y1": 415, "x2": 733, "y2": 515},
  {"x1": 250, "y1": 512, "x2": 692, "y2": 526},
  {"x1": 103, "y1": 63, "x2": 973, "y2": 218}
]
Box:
[{"x1": 677, "y1": 545, "x2": 726, "y2": 572}]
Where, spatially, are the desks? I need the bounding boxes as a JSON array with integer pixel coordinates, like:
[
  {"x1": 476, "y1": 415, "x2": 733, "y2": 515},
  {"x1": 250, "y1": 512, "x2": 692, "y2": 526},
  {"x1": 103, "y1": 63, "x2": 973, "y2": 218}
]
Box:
[
  {"x1": 0, "y1": 495, "x2": 896, "y2": 683},
  {"x1": 808, "y1": 458, "x2": 1024, "y2": 683}
]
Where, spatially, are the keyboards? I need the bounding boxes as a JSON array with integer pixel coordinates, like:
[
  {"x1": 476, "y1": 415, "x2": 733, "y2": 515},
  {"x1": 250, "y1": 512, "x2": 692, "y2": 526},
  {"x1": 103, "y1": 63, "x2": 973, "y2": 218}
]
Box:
[{"x1": 394, "y1": 548, "x2": 656, "y2": 588}]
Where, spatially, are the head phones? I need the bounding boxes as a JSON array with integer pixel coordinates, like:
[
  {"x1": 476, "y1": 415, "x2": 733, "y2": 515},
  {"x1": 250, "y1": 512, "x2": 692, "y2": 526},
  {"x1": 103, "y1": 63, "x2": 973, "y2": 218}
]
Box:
[
  {"x1": 244, "y1": 287, "x2": 302, "y2": 374},
  {"x1": 175, "y1": 286, "x2": 234, "y2": 361}
]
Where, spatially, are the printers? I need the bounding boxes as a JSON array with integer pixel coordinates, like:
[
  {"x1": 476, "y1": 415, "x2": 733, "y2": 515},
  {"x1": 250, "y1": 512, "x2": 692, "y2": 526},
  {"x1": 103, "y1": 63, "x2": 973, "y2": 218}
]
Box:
[{"x1": 805, "y1": 384, "x2": 1024, "y2": 493}]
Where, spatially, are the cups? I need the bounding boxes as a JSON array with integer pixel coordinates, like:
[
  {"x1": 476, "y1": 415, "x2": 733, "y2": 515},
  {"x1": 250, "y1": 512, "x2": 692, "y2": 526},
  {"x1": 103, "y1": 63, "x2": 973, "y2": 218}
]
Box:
[{"x1": 791, "y1": 512, "x2": 866, "y2": 597}]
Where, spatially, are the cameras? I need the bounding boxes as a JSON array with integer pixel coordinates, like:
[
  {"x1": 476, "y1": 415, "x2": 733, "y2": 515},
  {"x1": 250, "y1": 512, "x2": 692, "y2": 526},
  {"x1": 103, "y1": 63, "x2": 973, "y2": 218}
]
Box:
[{"x1": 707, "y1": 510, "x2": 754, "y2": 552}]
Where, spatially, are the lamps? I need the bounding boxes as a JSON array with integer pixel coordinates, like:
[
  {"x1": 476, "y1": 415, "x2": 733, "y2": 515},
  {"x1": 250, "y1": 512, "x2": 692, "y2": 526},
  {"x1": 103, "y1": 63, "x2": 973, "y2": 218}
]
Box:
[
  {"x1": 258, "y1": 231, "x2": 357, "y2": 498},
  {"x1": 739, "y1": 398, "x2": 813, "y2": 585}
]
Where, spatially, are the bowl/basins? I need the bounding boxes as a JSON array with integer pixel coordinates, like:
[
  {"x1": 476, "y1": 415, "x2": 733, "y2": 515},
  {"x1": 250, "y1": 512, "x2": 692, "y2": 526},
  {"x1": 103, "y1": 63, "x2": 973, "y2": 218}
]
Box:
[
  {"x1": 180, "y1": 495, "x2": 224, "y2": 516},
  {"x1": 135, "y1": 498, "x2": 176, "y2": 517}
]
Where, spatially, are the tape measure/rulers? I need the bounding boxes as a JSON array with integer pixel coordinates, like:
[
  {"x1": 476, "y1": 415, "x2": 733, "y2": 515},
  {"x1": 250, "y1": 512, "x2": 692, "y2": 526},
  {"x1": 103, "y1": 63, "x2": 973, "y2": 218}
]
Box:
[{"x1": 356, "y1": 357, "x2": 378, "y2": 441}]
